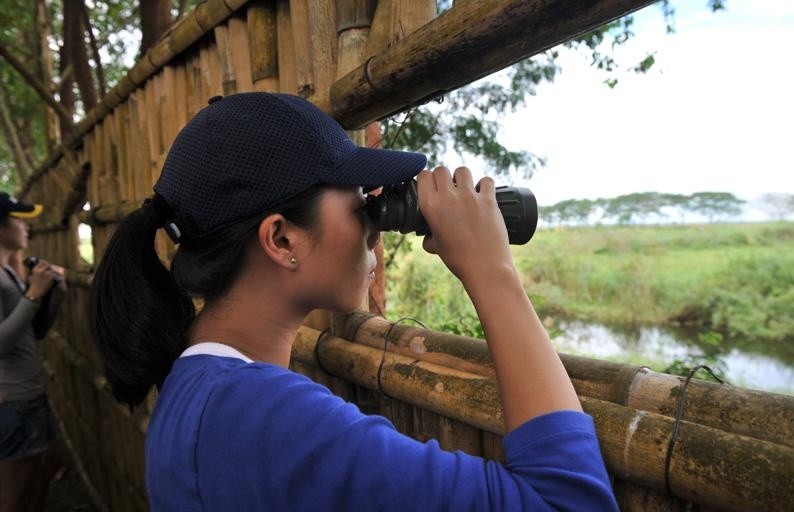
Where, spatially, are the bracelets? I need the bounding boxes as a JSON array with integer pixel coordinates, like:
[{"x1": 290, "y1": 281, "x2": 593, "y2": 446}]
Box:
[{"x1": 23, "y1": 295, "x2": 42, "y2": 304}]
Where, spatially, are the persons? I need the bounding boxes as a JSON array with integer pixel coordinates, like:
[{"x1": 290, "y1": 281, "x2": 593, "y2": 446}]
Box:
[
  {"x1": 87, "y1": 96, "x2": 620, "y2": 512},
  {"x1": 0, "y1": 190, "x2": 68, "y2": 511}
]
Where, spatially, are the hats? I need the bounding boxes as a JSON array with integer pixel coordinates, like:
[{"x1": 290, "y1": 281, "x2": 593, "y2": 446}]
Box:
[
  {"x1": 153, "y1": 91, "x2": 428, "y2": 245},
  {"x1": 1, "y1": 192, "x2": 44, "y2": 218}
]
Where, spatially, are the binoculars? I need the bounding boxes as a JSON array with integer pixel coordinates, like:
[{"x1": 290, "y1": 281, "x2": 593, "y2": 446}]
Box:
[
  {"x1": 23, "y1": 256, "x2": 61, "y2": 290},
  {"x1": 364, "y1": 172, "x2": 539, "y2": 246}
]
